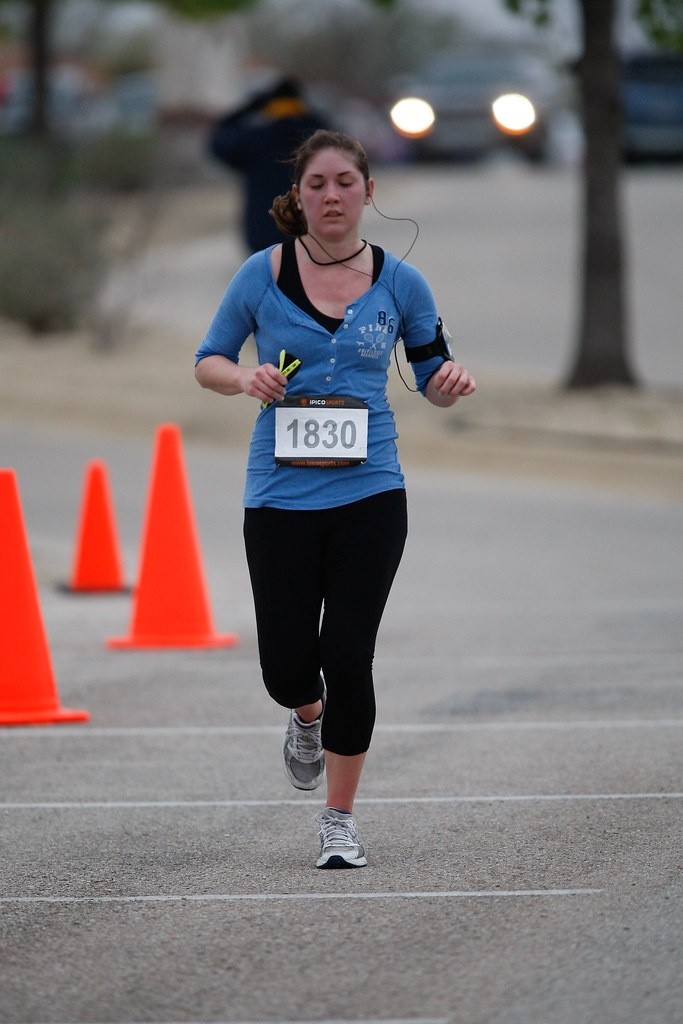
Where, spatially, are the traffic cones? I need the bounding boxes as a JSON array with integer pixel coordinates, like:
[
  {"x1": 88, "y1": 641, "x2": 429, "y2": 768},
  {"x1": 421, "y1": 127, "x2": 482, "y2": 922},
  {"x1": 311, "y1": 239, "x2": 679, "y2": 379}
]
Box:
[
  {"x1": 105, "y1": 421, "x2": 239, "y2": 650},
  {"x1": 55, "y1": 458, "x2": 130, "y2": 593},
  {"x1": 0, "y1": 467, "x2": 89, "y2": 727}
]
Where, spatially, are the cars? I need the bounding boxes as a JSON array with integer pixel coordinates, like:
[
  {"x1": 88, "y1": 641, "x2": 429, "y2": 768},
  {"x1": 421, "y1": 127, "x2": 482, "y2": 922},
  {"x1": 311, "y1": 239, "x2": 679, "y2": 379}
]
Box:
[{"x1": 391, "y1": 52, "x2": 551, "y2": 171}]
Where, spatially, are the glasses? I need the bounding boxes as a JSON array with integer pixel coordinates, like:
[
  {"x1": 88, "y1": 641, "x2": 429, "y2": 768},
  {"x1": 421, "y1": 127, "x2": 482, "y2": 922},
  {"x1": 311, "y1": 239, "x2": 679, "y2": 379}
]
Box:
[{"x1": 260, "y1": 349, "x2": 301, "y2": 406}]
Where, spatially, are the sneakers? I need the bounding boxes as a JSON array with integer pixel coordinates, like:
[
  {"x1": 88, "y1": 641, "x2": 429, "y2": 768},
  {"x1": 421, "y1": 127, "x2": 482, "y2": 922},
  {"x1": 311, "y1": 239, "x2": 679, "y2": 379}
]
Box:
[
  {"x1": 283, "y1": 687, "x2": 328, "y2": 791},
  {"x1": 317, "y1": 808, "x2": 369, "y2": 869}
]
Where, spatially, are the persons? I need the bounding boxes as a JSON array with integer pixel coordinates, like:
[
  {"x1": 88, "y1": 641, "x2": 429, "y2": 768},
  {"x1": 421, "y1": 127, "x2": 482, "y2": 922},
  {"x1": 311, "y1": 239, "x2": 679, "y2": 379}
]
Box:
[
  {"x1": 195, "y1": 130, "x2": 480, "y2": 867},
  {"x1": 208, "y1": 77, "x2": 336, "y2": 251}
]
